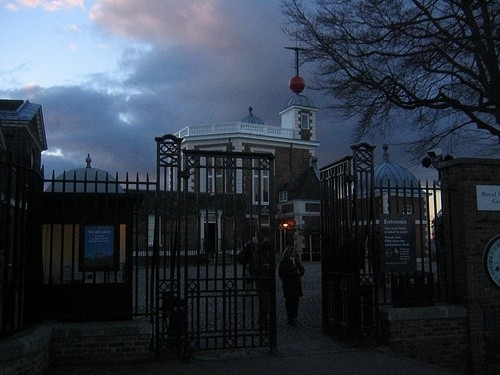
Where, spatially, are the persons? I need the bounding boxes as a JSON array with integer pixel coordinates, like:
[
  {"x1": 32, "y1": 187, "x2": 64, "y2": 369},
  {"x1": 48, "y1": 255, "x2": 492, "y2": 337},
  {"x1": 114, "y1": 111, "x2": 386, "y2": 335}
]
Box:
[
  {"x1": 236, "y1": 230, "x2": 273, "y2": 322},
  {"x1": 279, "y1": 245, "x2": 305, "y2": 329}
]
